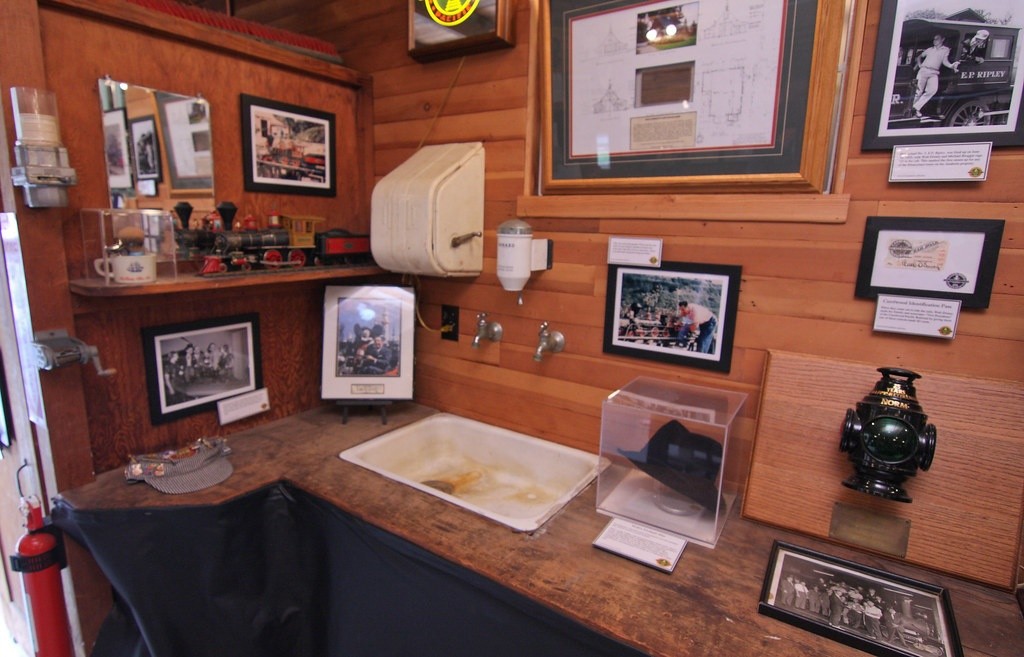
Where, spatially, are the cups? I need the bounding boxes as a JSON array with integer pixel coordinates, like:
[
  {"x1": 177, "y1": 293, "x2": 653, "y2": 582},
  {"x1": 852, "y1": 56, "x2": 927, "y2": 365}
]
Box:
[{"x1": 93, "y1": 252, "x2": 156, "y2": 284}]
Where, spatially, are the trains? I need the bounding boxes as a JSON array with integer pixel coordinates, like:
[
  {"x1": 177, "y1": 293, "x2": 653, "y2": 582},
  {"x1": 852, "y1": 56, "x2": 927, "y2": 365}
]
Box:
[
  {"x1": 889, "y1": 9, "x2": 1023, "y2": 127},
  {"x1": 172, "y1": 199, "x2": 371, "y2": 274},
  {"x1": 618, "y1": 308, "x2": 716, "y2": 354},
  {"x1": 880, "y1": 597, "x2": 946, "y2": 657}
]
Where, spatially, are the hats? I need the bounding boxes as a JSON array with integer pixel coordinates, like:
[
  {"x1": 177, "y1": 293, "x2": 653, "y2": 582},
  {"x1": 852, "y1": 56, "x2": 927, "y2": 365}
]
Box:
[
  {"x1": 975, "y1": 30, "x2": 989, "y2": 40},
  {"x1": 372, "y1": 324, "x2": 386, "y2": 340},
  {"x1": 618, "y1": 419, "x2": 726, "y2": 516},
  {"x1": 123, "y1": 434, "x2": 234, "y2": 495}
]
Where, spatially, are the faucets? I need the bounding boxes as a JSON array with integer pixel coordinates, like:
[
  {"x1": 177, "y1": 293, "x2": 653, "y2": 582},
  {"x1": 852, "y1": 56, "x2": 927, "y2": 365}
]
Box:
[
  {"x1": 532, "y1": 319, "x2": 565, "y2": 363},
  {"x1": 469, "y1": 311, "x2": 503, "y2": 349}
]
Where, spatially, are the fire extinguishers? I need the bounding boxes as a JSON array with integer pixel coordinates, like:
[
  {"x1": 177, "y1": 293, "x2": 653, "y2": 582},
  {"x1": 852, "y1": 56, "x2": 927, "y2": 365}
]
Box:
[{"x1": 16, "y1": 496, "x2": 76, "y2": 657}]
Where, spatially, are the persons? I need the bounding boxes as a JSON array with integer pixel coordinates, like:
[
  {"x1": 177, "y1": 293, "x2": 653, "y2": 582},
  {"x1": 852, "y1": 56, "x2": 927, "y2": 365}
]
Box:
[
  {"x1": 164, "y1": 343, "x2": 234, "y2": 404},
  {"x1": 961, "y1": 30, "x2": 990, "y2": 63},
  {"x1": 619, "y1": 301, "x2": 717, "y2": 353},
  {"x1": 912, "y1": 33, "x2": 960, "y2": 118},
  {"x1": 338, "y1": 324, "x2": 391, "y2": 374},
  {"x1": 781, "y1": 576, "x2": 910, "y2": 649}
]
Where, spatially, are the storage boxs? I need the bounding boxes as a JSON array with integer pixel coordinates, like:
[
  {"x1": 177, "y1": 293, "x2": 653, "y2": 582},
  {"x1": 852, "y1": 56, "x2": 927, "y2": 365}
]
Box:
[{"x1": 596, "y1": 375, "x2": 749, "y2": 547}]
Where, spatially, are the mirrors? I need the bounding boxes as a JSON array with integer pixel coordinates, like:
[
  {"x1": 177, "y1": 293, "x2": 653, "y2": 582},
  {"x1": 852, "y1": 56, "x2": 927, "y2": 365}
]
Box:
[{"x1": 98, "y1": 78, "x2": 216, "y2": 263}]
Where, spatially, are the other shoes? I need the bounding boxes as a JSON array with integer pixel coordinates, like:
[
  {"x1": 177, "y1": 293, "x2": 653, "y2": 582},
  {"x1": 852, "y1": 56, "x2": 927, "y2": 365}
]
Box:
[{"x1": 912, "y1": 108, "x2": 922, "y2": 118}]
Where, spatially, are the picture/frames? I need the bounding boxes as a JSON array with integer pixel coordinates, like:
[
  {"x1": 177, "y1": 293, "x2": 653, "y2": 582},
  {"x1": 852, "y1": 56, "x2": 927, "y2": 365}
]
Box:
[
  {"x1": 103, "y1": 107, "x2": 133, "y2": 191},
  {"x1": 151, "y1": 91, "x2": 214, "y2": 196},
  {"x1": 854, "y1": 216, "x2": 1005, "y2": 308},
  {"x1": 240, "y1": 92, "x2": 337, "y2": 198},
  {"x1": 860, "y1": 0, "x2": 1024, "y2": 152},
  {"x1": 601, "y1": 261, "x2": 743, "y2": 374},
  {"x1": 128, "y1": 114, "x2": 163, "y2": 181},
  {"x1": 139, "y1": 311, "x2": 264, "y2": 427},
  {"x1": 536, "y1": 0, "x2": 846, "y2": 196},
  {"x1": 318, "y1": 282, "x2": 416, "y2": 402},
  {"x1": 757, "y1": 539, "x2": 964, "y2": 657}
]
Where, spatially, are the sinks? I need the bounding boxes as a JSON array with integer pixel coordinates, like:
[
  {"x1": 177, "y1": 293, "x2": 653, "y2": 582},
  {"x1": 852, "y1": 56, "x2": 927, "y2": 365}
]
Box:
[{"x1": 339, "y1": 409, "x2": 613, "y2": 533}]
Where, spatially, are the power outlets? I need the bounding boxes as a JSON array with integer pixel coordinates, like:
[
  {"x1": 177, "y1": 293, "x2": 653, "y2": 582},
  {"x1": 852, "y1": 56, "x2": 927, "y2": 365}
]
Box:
[{"x1": 441, "y1": 304, "x2": 460, "y2": 341}]
